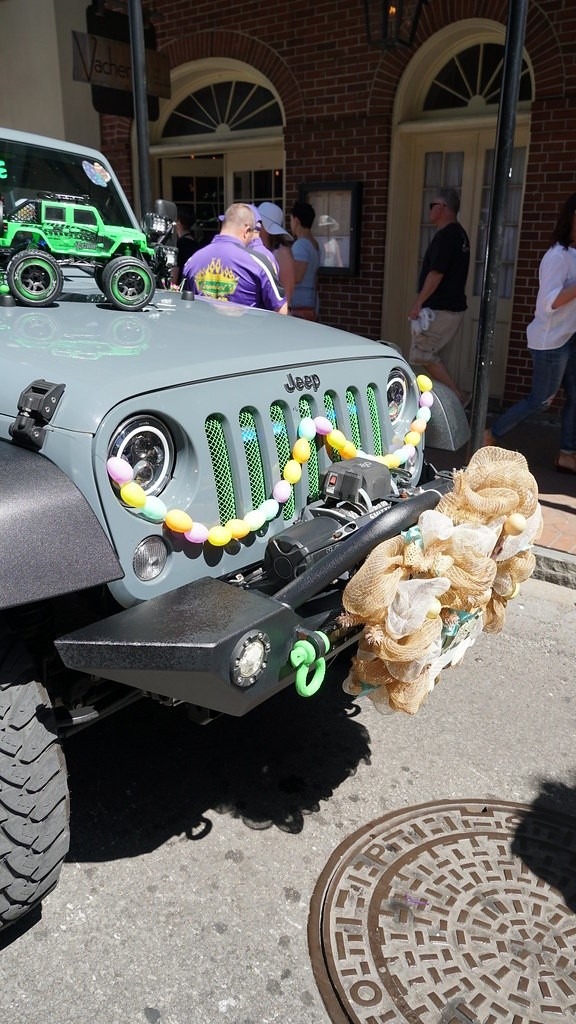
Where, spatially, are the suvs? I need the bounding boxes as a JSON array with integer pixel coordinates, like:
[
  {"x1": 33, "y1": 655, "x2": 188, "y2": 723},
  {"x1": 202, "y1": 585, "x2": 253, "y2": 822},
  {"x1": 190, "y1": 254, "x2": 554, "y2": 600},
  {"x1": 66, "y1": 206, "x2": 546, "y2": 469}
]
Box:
[
  {"x1": 0, "y1": 126, "x2": 473, "y2": 930},
  {"x1": 0, "y1": 190, "x2": 170, "y2": 311}
]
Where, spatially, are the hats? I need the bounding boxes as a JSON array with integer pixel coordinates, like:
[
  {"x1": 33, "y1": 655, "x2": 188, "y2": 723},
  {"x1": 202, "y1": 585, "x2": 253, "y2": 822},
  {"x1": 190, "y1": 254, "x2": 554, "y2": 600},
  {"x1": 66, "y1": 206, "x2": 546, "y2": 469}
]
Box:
[
  {"x1": 258, "y1": 201, "x2": 294, "y2": 241},
  {"x1": 219, "y1": 204, "x2": 263, "y2": 231}
]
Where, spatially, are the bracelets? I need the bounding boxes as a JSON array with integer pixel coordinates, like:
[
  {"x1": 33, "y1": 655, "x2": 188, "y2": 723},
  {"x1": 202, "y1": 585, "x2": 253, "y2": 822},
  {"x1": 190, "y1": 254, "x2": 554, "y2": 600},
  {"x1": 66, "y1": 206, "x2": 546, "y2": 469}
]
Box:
[
  {"x1": 288, "y1": 306, "x2": 295, "y2": 310},
  {"x1": 562, "y1": 289, "x2": 572, "y2": 298}
]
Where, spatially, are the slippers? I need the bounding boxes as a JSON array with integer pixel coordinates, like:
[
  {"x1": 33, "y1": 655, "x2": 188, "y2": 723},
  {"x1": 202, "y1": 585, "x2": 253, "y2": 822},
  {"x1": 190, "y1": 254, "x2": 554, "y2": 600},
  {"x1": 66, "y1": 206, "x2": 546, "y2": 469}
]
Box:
[{"x1": 462, "y1": 391, "x2": 473, "y2": 407}]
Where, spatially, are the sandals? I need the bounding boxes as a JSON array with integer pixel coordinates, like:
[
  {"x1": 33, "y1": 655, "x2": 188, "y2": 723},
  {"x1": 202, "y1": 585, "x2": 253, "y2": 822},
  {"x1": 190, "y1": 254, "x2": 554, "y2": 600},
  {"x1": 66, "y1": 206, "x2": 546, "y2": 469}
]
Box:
[{"x1": 555, "y1": 452, "x2": 576, "y2": 475}]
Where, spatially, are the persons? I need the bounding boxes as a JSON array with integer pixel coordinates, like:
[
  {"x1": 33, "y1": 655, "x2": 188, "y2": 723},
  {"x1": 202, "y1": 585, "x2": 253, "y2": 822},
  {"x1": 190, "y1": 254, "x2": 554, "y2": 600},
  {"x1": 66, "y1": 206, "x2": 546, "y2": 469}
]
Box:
[
  {"x1": 471, "y1": 191, "x2": 576, "y2": 476},
  {"x1": 288, "y1": 198, "x2": 321, "y2": 321},
  {"x1": 319, "y1": 215, "x2": 343, "y2": 267},
  {"x1": 408, "y1": 187, "x2": 473, "y2": 412},
  {"x1": 169, "y1": 212, "x2": 198, "y2": 287},
  {"x1": 183, "y1": 202, "x2": 296, "y2": 317}
]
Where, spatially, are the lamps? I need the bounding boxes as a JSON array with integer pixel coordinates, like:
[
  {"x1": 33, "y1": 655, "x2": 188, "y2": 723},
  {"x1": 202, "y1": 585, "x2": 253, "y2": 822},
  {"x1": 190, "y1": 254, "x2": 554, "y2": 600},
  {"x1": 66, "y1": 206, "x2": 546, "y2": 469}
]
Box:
[{"x1": 363, "y1": 0, "x2": 424, "y2": 50}]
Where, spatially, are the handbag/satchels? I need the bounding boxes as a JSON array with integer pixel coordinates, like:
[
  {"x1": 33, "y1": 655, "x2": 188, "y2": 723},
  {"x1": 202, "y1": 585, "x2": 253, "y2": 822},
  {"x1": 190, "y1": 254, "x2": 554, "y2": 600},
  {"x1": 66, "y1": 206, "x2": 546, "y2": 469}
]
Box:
[{"x1": 290, "y1": 306, "x2": 315, "y2": 322}]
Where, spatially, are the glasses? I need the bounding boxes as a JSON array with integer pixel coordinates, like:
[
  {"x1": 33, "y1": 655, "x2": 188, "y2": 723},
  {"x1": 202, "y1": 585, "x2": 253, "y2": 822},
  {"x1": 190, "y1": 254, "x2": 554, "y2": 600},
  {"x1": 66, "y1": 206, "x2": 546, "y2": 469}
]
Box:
[{"x1": 429, "y1": 203, "x2": 447, "y2": 210}]
